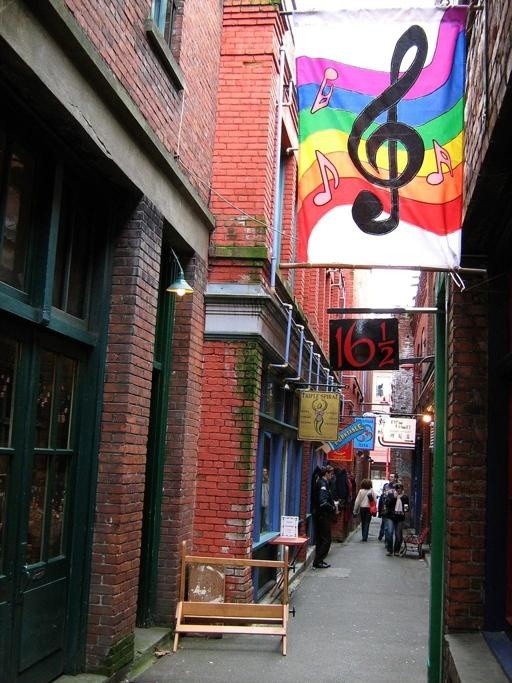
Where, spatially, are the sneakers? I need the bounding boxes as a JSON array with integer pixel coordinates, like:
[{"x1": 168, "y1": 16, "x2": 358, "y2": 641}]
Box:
[
  {"x1": 394, "y1": 552, "x2": 399, "y2": 556},
  {"x1": 385, "y1": 552, "x2": 393, "y2": 556}
]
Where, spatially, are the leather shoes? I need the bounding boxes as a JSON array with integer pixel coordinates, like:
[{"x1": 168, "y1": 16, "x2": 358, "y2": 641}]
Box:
[{"x1": 313, "y1": 562, "x2": 330, "y2": 568}]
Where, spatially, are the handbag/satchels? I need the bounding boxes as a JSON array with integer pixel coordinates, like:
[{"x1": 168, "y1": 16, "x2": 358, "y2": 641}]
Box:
[{"x1": 369, "y1": 489, "x2": 377, "y2": 517}]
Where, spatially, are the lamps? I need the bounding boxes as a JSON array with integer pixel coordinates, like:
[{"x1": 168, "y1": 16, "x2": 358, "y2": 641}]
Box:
[{"x1": 166, "y1": 244, "x2": 195, "y2": 297}]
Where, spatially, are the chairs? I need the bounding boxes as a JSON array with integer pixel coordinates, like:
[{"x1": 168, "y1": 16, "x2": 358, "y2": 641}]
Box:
[{"x1": 400, "y1": 526, "x2": 430, "y2": 557}]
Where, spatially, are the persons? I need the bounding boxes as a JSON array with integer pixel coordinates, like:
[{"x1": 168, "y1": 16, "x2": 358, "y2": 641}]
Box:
[
  {"x1": 313, "y1": 464, "x2": 337, "y2": 568},
  {"x1": 377, "y1": 483, "x2": 389, "y2": 542},
  {"x1": 385, "y1": 473, "x2": 402, "y2": 548},
  {"x1": 381, "y1": 484, "x2": 411, "y2": 557},
  {"x1": 262, "y1": 467, "x2": 271, "y2": 534},
  {"x1": 353, "y1": 479, "x2": 378, "y2": 543}
]
